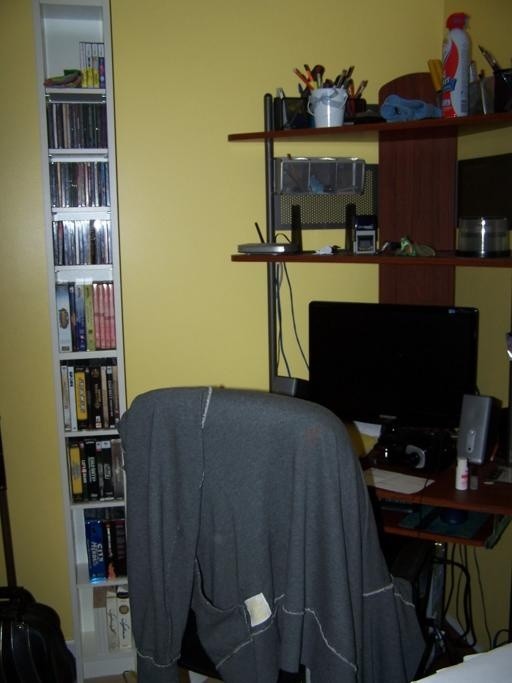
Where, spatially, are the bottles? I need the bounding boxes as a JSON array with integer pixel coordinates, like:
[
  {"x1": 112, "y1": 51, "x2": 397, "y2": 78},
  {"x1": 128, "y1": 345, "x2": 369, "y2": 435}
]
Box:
[{"x1": 456, "y1": 456, "x2": 469, "y2": 491}]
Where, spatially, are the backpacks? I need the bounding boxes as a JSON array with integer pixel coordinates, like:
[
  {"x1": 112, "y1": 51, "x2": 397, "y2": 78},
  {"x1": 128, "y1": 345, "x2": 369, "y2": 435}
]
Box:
[{"x1": 1, "y1": 584, "x2": 77, "y2": 683}]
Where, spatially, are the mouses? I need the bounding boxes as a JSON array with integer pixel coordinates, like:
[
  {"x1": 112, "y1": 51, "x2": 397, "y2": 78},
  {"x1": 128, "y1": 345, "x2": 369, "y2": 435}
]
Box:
[{"x1": 441, "y1": 509, "x2": 469, "y2": 525}]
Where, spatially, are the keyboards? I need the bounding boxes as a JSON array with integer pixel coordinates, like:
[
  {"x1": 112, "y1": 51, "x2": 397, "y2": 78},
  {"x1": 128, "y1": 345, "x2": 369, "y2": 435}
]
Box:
[
  {"x1": 363, "y1": 466, "x2": 436, "y2": 494},
  {"x1": 369, "y1": 497, "x2": 414, "y2": 514}
]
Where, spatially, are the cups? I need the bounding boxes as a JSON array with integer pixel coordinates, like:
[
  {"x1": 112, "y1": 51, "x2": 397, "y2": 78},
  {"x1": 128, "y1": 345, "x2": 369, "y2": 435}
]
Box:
[{"x1": 468, "y1": 65, "x2": 512, "y2": 115}]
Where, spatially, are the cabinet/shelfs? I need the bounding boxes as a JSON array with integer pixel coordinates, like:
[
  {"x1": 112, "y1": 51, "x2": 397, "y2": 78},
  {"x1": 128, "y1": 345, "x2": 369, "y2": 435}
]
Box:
[
  {"x1": 225, "y1": 70, "x2": 511, "y2": 268},
  {"x1": 38, "y1": 1, "x2": 132, "y2": 683}
]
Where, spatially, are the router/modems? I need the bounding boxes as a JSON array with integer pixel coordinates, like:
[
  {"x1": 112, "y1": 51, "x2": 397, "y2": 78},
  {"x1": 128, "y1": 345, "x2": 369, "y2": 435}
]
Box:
[{"x1": 236, "y1": 222, "x2": 296, "y2": 256}]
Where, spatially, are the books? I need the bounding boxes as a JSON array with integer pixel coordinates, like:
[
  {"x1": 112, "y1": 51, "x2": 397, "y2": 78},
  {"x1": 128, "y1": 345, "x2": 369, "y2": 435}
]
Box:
[
  {"x1": 79, "y1": 41, "x2": 105, "y2": 88},
  {"x1": 60, "y1": 364, "x2": 125, "y2": 503},
  {"x1": 45, "y1": 103, "x2": 110, "y2": 207},
  {"x1": 52, "y1": 219, "x2": 117, "y2": 352},
  {"x1": 69, "y1": 507, "x2": 133, "y2": 654}
]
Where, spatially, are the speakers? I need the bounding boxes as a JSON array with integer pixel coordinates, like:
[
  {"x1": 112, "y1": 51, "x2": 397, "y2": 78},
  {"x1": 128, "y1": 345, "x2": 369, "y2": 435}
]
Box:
[
  {"x1": 270, "y1": 375, "x2": 307, "y2": 400},
  {"x1": 458, "y1": 395, "x2": 501, "y2": 465}
]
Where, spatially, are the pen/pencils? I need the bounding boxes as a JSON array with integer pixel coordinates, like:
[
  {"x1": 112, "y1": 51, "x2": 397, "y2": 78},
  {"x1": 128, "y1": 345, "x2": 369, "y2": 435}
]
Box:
[
  {"x1": 477, "y1": 44, "x2": 509, "y2": 87},
  {"x1": 292, "y1": 63, "x2": 367, "y2": 120}
]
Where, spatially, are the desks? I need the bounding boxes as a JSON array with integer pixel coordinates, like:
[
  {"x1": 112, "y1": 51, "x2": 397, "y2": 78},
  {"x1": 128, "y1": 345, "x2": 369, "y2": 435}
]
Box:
[{"x1": 348, "y1": 421, "x2": 511, "y2": 676}]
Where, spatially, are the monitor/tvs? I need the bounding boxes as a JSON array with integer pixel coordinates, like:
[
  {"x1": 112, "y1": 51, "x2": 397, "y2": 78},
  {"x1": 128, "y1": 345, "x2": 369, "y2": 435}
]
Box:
[{"x1": 308, "y1": 300, "x2": 479, "y2": 474}]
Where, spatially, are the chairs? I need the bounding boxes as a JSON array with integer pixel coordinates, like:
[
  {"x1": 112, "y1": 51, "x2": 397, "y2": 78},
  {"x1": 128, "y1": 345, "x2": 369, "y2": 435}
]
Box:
[{"x1": 116, "y1": 385, "x2": 420, "y2": 682}]
